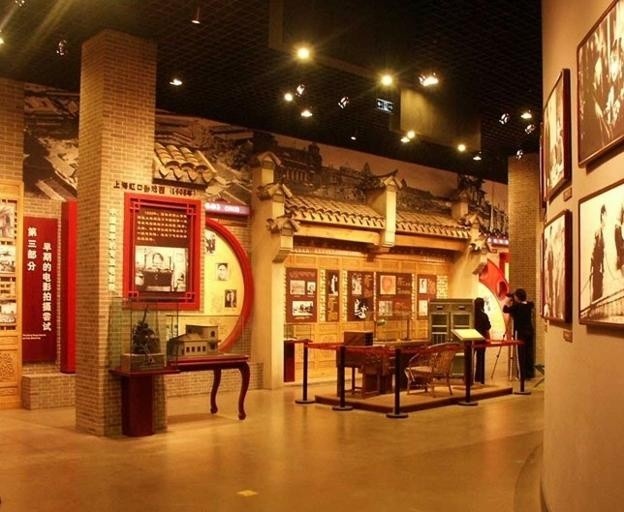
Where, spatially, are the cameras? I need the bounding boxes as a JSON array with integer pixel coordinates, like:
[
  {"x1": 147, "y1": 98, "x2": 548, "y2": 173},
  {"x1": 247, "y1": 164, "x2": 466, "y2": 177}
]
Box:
[{"x1": 505, "y1": 293, "x2": 514, "y2": 302}]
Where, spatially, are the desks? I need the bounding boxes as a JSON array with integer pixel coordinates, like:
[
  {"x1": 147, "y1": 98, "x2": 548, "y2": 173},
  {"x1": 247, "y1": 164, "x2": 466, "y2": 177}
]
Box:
[{"x1": 167, "y1": 351, "x2": 250, "y2": 420}]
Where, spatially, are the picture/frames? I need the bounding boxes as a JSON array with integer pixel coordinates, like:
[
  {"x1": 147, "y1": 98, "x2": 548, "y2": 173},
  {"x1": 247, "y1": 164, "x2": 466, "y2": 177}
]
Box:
[{"x1": 541, "y1": 2, "x2": 623, "y2": 330}]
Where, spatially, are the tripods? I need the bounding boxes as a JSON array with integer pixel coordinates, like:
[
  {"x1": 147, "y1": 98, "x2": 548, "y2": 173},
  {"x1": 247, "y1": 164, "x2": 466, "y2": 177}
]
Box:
[{"x1": 490, "y1": 302, "x2": 519, "y2": 381}]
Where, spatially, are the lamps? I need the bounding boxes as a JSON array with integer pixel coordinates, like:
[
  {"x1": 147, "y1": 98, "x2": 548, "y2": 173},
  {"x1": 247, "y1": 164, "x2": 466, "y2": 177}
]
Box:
[
  {"x1": 192, "y1": 6, "x2": 201, "y2": 24},
  {"x1": 285, "y1": 35, "x2": 535, "y2": 160},
  {"x1": 56, "y1": 39, "x2": 68, "y2": 56},
  {"x1": 169, "y1": 67, "x2": 185, "y2": 86}
]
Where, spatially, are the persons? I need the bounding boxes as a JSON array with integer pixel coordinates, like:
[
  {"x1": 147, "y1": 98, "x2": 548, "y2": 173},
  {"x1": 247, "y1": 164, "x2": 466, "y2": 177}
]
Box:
[
  {"x1": 224, "y1": 289, "x2": 236, "y2": 308},
  {"x1": 216, "y1": 263, "x2": 228, "y2": 280},
  {"x1": 474, "y1": 297, "x2": 490, "y2": 384},
  {"x1": 541, "y1": 29, "x2": 624, "y2": 331},
  {"x1": 502, "y1": 289, "x2": 536, "y2": 380}
]
